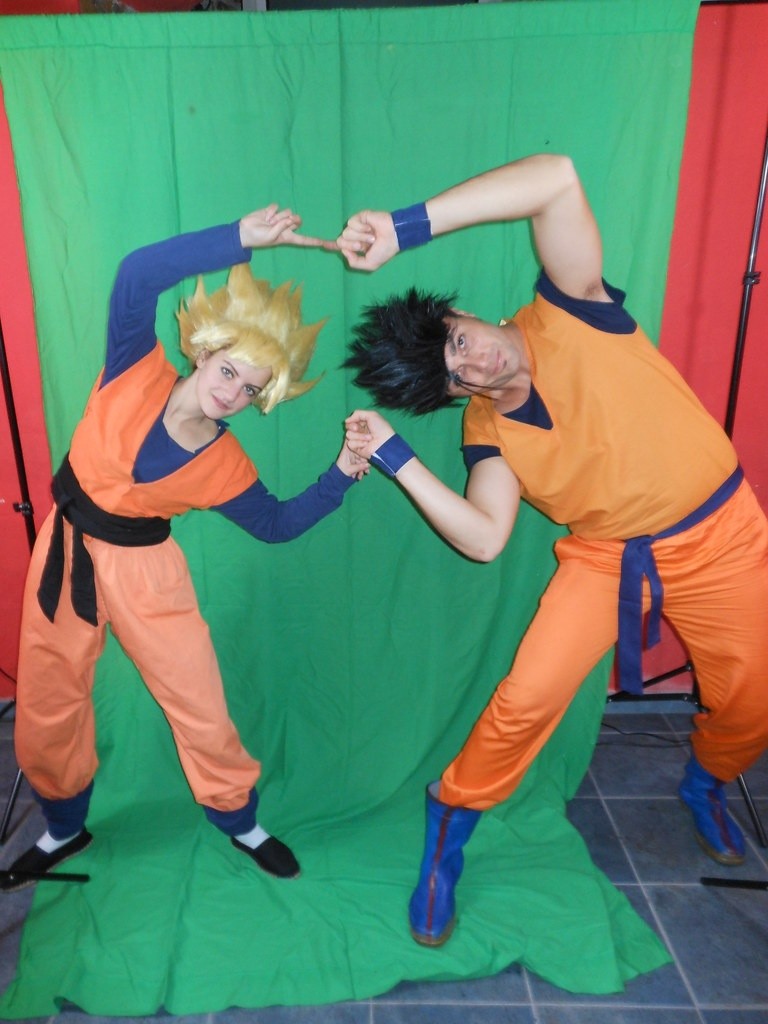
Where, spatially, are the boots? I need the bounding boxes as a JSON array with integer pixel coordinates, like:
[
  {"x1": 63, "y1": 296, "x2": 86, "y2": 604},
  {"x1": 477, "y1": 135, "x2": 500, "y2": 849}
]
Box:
[
  {"x1": 676, "y1": 752, "x2": 749, "y2": 865},
  {"x1": 408, "y1": 781, "x2": 483, "y2": 945}
]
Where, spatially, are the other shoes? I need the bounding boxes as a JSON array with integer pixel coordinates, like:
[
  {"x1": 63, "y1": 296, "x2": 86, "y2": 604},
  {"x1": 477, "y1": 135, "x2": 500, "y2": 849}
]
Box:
[
  {"x1": 0, "y1": 827, "x2": 93, "y2": 892},
  {"x1": 231, "y1": 834, "x2": 301, "y2": 879}
]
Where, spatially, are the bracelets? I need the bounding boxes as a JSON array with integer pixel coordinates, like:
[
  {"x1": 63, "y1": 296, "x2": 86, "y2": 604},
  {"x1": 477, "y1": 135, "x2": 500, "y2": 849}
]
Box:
[
  {"x1": 389, "y1": 202, "x2": 433, "y2": 251},
  {"x1": 367, "y1": 432, "x2": 418, "y2": 480}
]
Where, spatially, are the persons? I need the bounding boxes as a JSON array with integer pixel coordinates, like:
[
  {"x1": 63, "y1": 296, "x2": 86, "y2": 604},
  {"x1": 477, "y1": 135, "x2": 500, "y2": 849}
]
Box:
[
  {"x1": 5, "y1": 205, "x2": 371, "y2": 891},
  {"x1": 322, "y1": 151, "x2": 768, "y2": 947}
]
